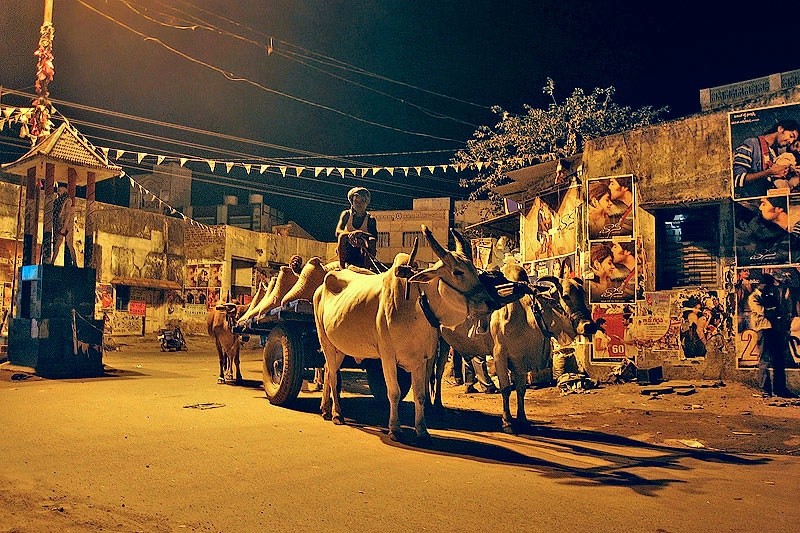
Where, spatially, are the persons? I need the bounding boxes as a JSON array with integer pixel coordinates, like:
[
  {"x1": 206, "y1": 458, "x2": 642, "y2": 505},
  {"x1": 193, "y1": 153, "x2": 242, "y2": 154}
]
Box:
[
  {"x1": 289, "y1": 254, "x2": 302, "y2": 273},
  {"x1": 335, "y1": 188, "x2": 379, "y2": 271},
  {"x1": 747, "y1": 273, "x2": 791, "y2": 398},
  {"x1": 452, "y1": 264, "x2": 527, "y2": 395},
  {"x1": 677, "y1": 268, "x2": 759, "y2": 363},
  {"x1": 182, "y1": 262, "x2": 220, "y2": 312},
  {"x1": 734, "y1": 116, "x2": 799, "y2": 266},
  {"x1": 532, "y1": 173, "x2": 636, "y2": 377}
]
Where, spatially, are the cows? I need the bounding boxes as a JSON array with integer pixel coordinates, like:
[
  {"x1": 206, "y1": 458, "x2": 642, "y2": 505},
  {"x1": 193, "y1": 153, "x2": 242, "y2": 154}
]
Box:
[{"x1": 312, "y1": 225, "x2": 496, "y2": 450}]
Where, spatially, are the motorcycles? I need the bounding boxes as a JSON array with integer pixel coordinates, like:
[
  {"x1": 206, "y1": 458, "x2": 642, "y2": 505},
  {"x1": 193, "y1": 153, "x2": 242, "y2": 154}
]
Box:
[{"x1": 155, "y1": 319, "x2": 188, "y2": 352}]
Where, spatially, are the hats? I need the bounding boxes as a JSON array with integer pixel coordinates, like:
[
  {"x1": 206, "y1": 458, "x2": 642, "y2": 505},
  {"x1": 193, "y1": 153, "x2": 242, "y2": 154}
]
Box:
[{"x1": 347, "y1": 186, "x2": 371, "y2": 204}]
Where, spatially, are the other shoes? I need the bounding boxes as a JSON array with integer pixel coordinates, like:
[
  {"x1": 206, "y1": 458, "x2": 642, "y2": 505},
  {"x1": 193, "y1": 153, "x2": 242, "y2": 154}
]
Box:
[{"x1": 763, "y1": 392, "x2": 770, "y2": 398}]
